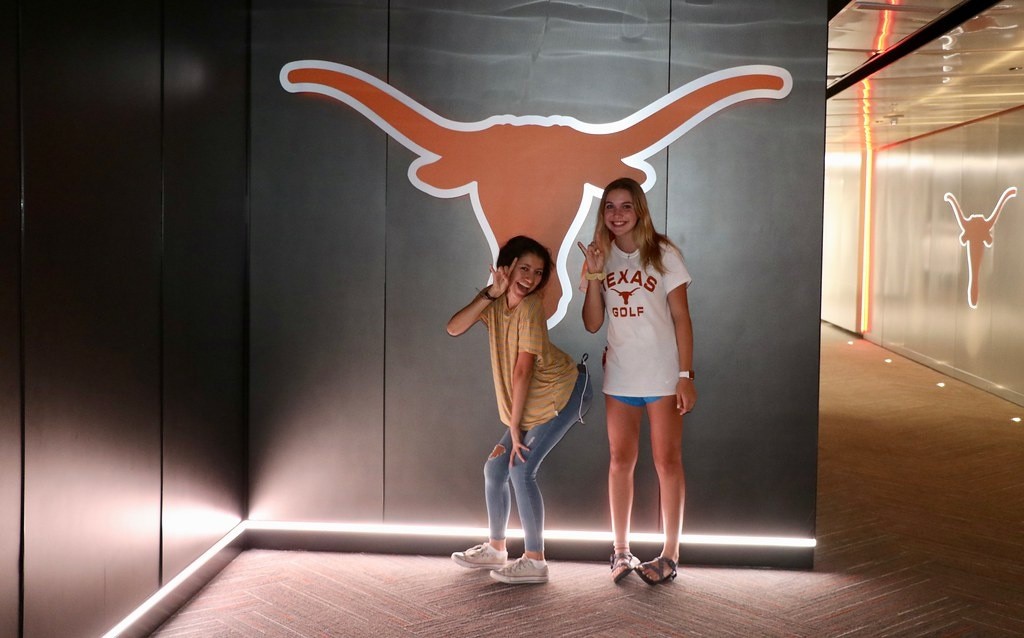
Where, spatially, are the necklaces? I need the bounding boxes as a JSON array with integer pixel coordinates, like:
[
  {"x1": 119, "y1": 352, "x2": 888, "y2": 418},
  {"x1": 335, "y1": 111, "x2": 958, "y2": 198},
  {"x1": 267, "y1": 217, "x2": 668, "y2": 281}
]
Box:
[{"x1": 619, "y1": 248, "x2": 639, "y2": 258}]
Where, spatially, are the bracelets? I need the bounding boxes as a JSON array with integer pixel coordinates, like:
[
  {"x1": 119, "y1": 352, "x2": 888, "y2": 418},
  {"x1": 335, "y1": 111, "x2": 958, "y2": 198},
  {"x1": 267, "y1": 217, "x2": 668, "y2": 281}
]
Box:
[
  {"x1": 479, "y1": 285, "x2": 497, "y2": 302},
  {"x1": 585, "y1": 271, "x2": 606, "y2": 280}
]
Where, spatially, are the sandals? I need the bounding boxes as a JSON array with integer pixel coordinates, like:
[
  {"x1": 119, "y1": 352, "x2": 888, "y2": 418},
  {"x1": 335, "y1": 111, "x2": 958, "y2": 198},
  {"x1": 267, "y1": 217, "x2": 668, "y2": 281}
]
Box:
[
  {"x1": 610, "y1": 550, "x2": 644, "y2": 586},
  {"x1": 634, "y1": 552, "x2": 679, "y2": 586}
]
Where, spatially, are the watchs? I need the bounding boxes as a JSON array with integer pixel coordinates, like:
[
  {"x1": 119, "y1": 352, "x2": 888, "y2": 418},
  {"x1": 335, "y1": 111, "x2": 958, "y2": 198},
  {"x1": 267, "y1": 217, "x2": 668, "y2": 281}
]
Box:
[{"x1": 679, "y1": 370, "x2": 695, "y2": 380}]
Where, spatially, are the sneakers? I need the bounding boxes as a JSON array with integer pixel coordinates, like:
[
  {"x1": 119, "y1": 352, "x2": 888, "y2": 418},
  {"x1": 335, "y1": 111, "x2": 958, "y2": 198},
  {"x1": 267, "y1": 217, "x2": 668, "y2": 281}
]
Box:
[
  {"x1": 451, "y1": 543, "x2": 515, "y2": 574},
  {"x1": 490, "y1": 553, "x2": 549, "y2": 583}
]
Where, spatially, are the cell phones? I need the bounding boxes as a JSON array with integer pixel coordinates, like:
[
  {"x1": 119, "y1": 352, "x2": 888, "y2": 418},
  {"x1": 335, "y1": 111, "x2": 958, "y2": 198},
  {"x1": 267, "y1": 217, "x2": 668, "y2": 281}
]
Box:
[{"x1": 577, "y1": 364, "x2": 587, "y2": 373}]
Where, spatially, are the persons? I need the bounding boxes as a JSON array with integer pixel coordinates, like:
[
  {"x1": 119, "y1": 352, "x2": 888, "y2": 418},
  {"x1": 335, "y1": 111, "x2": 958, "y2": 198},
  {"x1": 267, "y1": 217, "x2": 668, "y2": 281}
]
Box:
[
  {"x1": 578, "y1": 177, "x2": 698, "y2": 586},
  {"x1": 446, "y1": 236, "x2": 593, "y2": 584}
]
